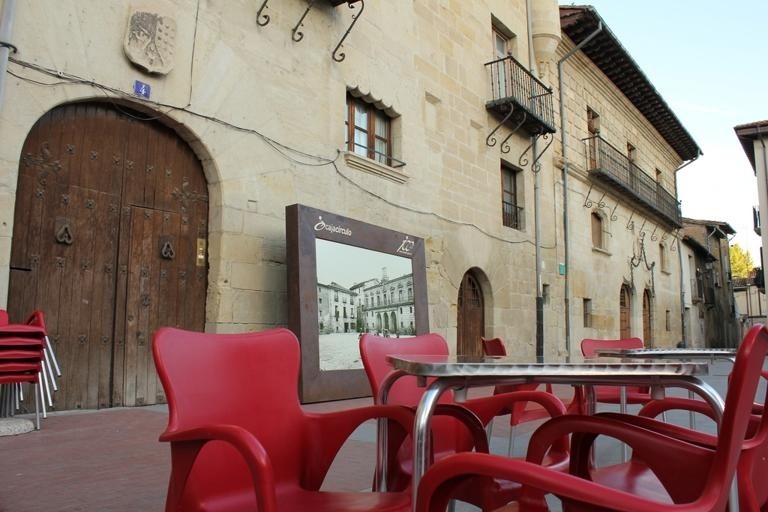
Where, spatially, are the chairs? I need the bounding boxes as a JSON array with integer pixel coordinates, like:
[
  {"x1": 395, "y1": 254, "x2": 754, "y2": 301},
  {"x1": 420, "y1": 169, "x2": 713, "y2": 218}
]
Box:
[
  {"x1": 359, "y1": 333, "x2": 570, "y2": 512},
  {"x1": 581, "y1": 337, "x2": 667, "y2": 464},
  {"x1": 0, "y1": 309, "x2": 62, "y2": 431},
  {"x1": 631, "y1": 369, "x2": 768, "y2": 512},
  {"x1": 480, "y1": 337, "x2": 588, "y2": 458},
  {"x1": 152, "y1": 326, "x2": 433, "y2": 512},
  {"x1": 416, "y1": 323, "x2": 768, "y2": 512}
]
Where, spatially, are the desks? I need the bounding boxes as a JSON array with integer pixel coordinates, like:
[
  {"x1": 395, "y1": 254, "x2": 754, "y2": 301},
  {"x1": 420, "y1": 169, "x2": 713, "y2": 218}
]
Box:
[
  {"x1": 378, "y1": 353, "x2": 740, "y2": 512},
  {"x1": 594, "y1": 347, "x2": 738, "y2": 463}
]
